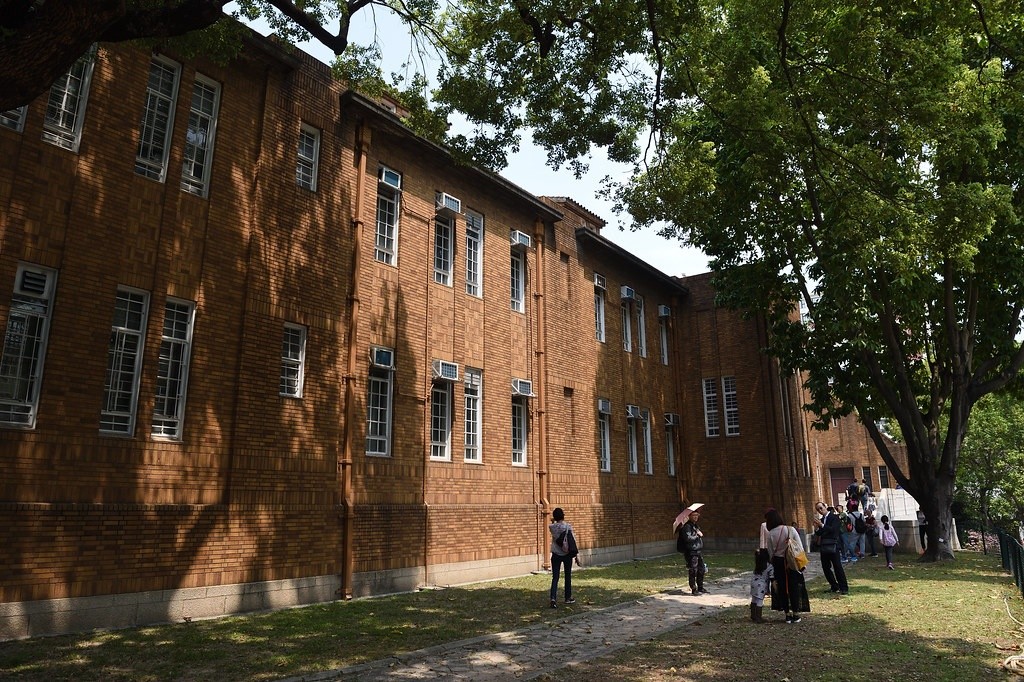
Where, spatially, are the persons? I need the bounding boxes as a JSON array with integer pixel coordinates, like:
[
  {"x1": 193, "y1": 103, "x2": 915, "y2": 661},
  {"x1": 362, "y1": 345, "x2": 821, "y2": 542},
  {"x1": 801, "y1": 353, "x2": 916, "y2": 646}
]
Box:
[
  {"x1": 549, "y1": 508, "x2": 582, "y2": 608},
  {"x1": 834, "y1": 505, "x2": 858, "y2": 562},
  {"x1": 865, "y1": 509, "x2": 880, "y2": 558},
  {"x1": 766, "y1": 512, "x2": 811, "y2": 624},
  {"x1": 750, "y1": 548, "x2": 777, "y2": 624},
  {"x1": 846, "y1": 494, "x2": 860, "y2": 514},
  {"x1": 859, "y1": 479, "x2": 875, "y2": 516},
  {"x1": 677, "y1": 510, "x2": 710, "y2": 596},
  {"x1": 916, "y1": 506, "x2": 929, "y2": 555},
  {"x1": 813, "y1": 502, "x2": 848, "y2": 595},
  {"x1": 879, "y1": 515, "x2": 900, "y2": 570},
  {"x1": 895, "y1": 481, "x2": 902, "y2": 489},
  {"x1": 847, "y1": 506, "x2": 866, "y2": 562},
  {"x1": 845, "y1": 479, "x2": 861, "y2": 506},
  {"x1": 792, "y1": 522, "x2": 800, "y2": 536}
]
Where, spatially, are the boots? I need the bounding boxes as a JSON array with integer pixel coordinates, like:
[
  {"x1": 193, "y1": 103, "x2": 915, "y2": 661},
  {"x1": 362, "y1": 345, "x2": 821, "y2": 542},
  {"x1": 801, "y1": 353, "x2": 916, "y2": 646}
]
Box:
[
  {"x1": 755, "y1": 606, "x2": 769, "y2": 623},
  {"x1": 750, "y1": 603, "x2": 757, "y2": 622}
]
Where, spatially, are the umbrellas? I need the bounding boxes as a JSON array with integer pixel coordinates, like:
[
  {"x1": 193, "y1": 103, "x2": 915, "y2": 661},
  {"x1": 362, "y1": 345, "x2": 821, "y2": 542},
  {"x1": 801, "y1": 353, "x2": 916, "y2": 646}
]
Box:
[{"x1": 673, "y1": 502, "x2": 705, "y2": 537}]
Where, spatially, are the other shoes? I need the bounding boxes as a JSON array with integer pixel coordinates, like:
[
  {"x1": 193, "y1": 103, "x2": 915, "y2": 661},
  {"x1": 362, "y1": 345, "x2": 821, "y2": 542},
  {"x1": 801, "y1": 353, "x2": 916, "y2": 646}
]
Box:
[
  {"x1": 840, "y1": 557, "x2": 848, "y2": 563},
  {"x1": 861, "y1": 554, "x2": 865, "y2": 558},
  {"x1": 840, "y1": 589, "x2": 848, "y2": 594},
  {"x1": 793, "y1": 615, "x2": 801, "y2": 622},
  {"x1": 886, "y1": 563, "x2": 894, "y2": 570},
  {"x1": 824, "y1": 587, "x2": 839, "y2": 593},
  {"x1": 692, "y1": 589, "x2": 703, "y2": 595},
  {"x1": 785, "y1": 616, "x2": 792, "y2": 623},
  {"x1": 850, "y1": 556, "x2": 857, "y2": 561},
  {"x1": 698, "y1": 587, "x2": 710, "y2": 593},
  {"x1": 550, "y1": 599, "x2": 557, "y2": 608},
  {"x1": 565, "y1": 598, "x2": 575, "y2": 604},
  {"x1": 868, "y1": 553, "x2": 878, "y2": 557}
]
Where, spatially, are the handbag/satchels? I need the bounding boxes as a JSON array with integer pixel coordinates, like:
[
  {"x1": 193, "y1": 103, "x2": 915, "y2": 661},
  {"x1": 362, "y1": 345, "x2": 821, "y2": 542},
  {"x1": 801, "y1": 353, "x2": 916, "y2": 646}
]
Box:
[
  {"x1": 784, "y1": 526, "x2": 809, "y2": 571},
  {"x1": 562, "y1": 523, "x2": 569, "y2": 553},
  {"x1": 821, "y1": 539, "x2": 837, "y2": 553}
]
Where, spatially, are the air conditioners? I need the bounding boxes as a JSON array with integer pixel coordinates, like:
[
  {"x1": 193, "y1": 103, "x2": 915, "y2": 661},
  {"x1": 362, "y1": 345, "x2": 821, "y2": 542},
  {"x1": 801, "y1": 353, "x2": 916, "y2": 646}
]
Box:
[
  {"x1": 658, "y1": 305, "x2": 671, "y2": 318},
  {"x1": 664, "y1": 413, "x2": 680, "y2": 425},
  {"x1": 512, "y1": 379, "x2": 538, "y2": 397},
  {"x1": 432, "y1": 360, "x2": 458, "y2": 381},
  {"x1": 435, "y1": 192, "x2": 461, "y2": 220},
  {"x1": 594, "y1": 273, "x2": 606, "y2": 291},
  {"x1": 378, "y1": 167, "x2": 401, "y2": 193},
  {"x1": 626, "y1": 405, "x2": 644, "y2": 419},
  {"x1": 510, "y1": 230, "x2": 531, "y2": 251},
  {"x1": 369, "y1": 348, "x2": 394, "y2": 370},
  {"x1": 621, "y1": 286, "x2": 635, "y2": 301},
  {"x1": 598, "y1": 399, "x2": 611, "y2": 414}
]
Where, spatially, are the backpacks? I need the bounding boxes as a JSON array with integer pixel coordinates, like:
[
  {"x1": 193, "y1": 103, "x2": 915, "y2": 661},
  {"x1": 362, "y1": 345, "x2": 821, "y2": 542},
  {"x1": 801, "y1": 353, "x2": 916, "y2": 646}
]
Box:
[
  {"x1": 880, "y1": 526, "x2": 896, "y2": 546},
  {"x1": 841, "y1": 514, "x2": 853, "y2": 532},
  {"x1": 676, "y1": 522, "x2": 694, "y2": 553},
  {"x1": 851, "y1": 512, "x2": 865, "y2": 533}
]
